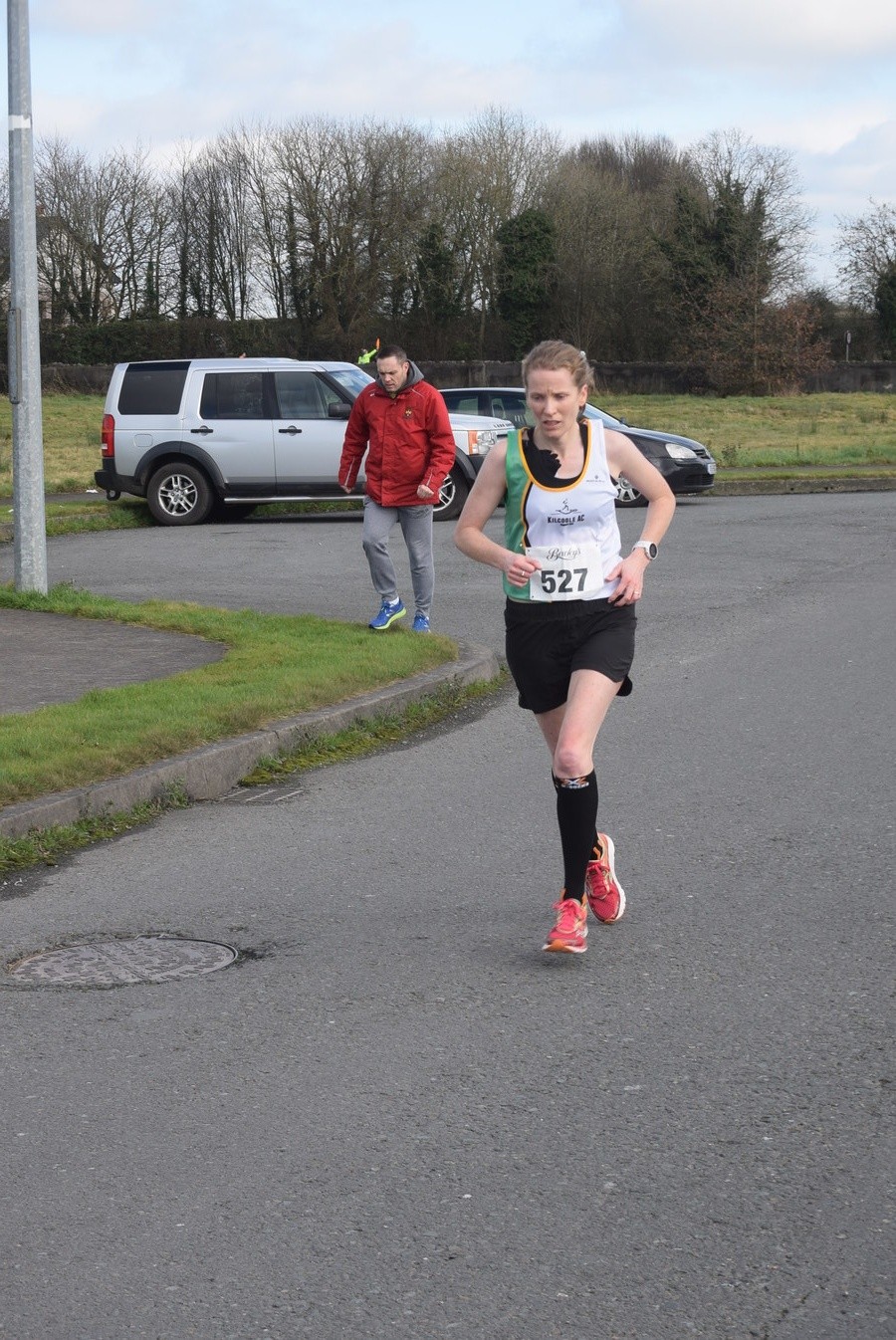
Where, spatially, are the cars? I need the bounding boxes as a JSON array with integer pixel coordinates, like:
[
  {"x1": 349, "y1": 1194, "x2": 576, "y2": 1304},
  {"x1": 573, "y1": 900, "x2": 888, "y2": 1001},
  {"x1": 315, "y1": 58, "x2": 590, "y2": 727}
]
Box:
[{"x1": 436, "y1": 387, "x2": 716, "y2": 508}]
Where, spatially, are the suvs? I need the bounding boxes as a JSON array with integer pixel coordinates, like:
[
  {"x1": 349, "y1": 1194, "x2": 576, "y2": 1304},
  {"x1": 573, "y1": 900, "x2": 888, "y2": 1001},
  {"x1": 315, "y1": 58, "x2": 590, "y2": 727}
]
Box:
[{"x1": 93, "y1": 357, "x2": 515, "y2": 525}]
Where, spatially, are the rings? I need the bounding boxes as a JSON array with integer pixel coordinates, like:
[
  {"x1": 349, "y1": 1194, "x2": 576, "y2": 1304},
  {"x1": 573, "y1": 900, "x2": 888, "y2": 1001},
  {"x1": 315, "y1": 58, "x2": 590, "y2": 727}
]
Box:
[
  {"x1": 520, "y1": 569, "x2": 525, "y2": 577},
  {"x1": 633, "y1": 592, "x2": 640, "y2": 596}
]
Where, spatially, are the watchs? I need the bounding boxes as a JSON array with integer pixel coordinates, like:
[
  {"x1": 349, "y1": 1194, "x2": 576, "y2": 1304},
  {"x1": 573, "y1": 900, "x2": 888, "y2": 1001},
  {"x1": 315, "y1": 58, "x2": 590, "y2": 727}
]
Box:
[{"x1": 631, "y1": 541, "x2": 659, "y2": 561}]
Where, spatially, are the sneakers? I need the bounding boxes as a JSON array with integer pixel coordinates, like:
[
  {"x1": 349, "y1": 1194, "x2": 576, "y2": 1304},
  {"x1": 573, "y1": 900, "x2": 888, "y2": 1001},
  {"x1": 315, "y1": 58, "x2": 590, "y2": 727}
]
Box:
[
  {"x1": 412, "y1": 615, "x2": 431, "y2": 634},
  {"x1": 587, "y1": 833, "x2": 625, "y2": 923},
  {"x1": 368, "y1": 598, "x2": 407, "y2": 630},
  {"x1": 541, "y1": 888, "x2": 589, "y2": 953}
]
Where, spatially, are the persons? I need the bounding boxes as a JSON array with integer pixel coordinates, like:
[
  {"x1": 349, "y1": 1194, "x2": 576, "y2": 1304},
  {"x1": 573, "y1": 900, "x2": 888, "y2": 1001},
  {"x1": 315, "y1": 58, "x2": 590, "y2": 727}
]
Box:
[
  {"x1": 450, "y1": 339, "x2": 678, "y2": 957},
  {"x1": 337, "y1": 344, "x2": 456, "y2": 636}
]
